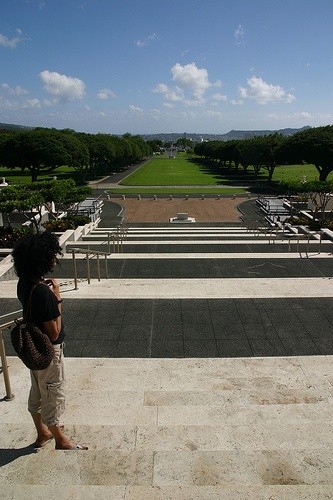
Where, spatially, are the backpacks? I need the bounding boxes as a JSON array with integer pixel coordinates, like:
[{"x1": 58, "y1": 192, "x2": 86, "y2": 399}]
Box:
[{"x1": 11, "y1": 319, "x2": 55, "y2": 370}]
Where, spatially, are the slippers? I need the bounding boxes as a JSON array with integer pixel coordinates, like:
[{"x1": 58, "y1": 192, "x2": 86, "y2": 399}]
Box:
[
  {"x1": 55, "y1": 445, "x2": 88, "y2": 450},
  {"x1": 36, "y1": 424, "x2": 65, "y2": 447}
]
